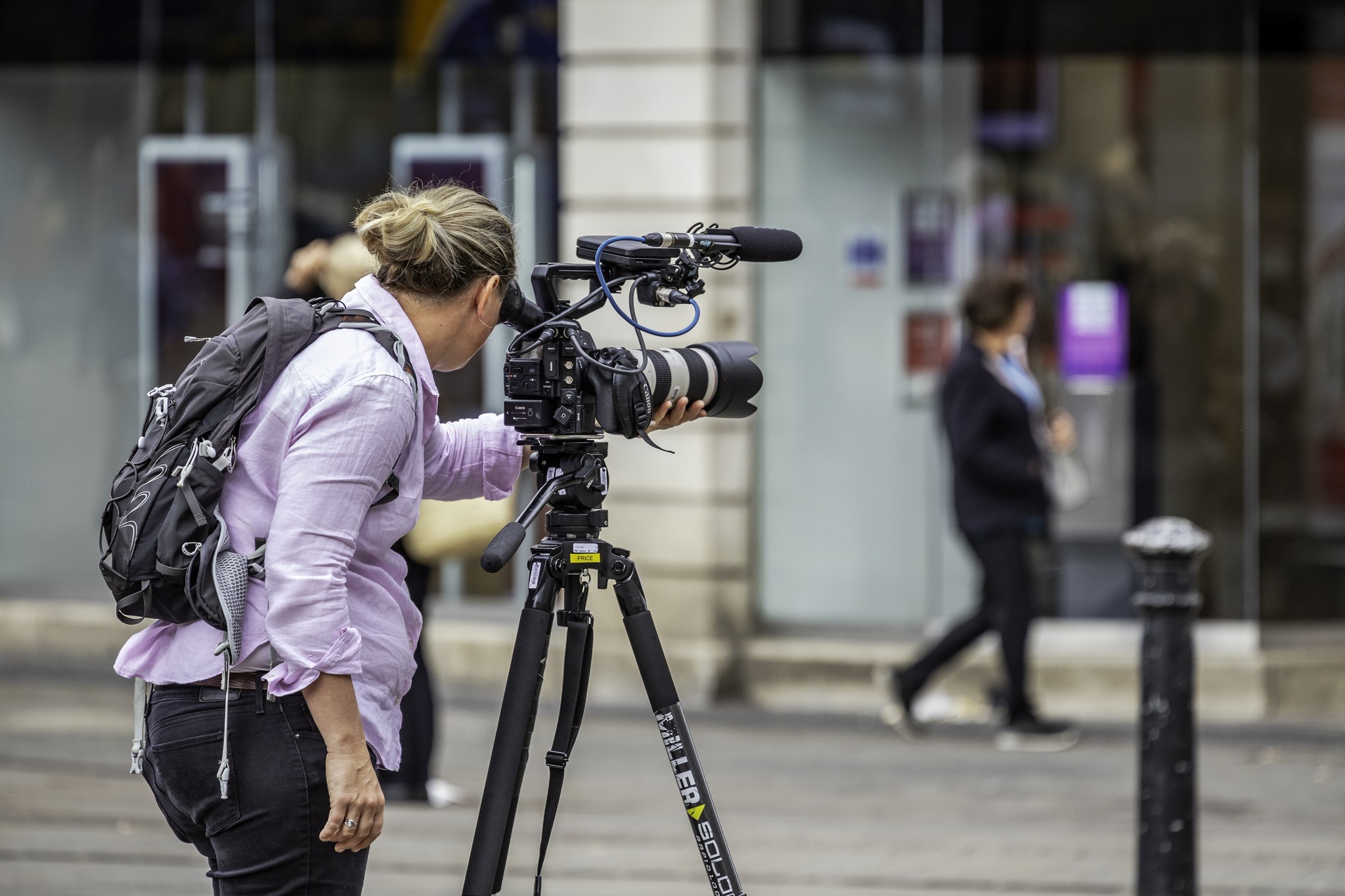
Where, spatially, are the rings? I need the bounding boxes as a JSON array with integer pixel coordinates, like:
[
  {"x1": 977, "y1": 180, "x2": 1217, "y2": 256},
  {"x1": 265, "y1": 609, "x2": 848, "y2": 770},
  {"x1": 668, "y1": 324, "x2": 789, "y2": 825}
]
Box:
[{"x1": 342, "y1": 819, "x2": 359, "y2": 828}]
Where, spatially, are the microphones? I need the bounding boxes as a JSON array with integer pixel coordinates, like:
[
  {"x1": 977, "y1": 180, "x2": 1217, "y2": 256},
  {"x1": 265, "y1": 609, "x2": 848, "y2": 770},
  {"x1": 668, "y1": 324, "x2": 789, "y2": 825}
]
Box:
[{"x1": 642, "y1": 227, "x2": 800, "y2": 262}]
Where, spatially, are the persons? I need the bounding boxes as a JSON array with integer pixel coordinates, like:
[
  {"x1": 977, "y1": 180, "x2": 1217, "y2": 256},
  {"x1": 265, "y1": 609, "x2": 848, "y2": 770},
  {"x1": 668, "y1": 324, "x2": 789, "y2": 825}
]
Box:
[
  {"x1": 115, "y1": 184, "x2": 707, "y2": 896},
  {"x1": 872, "y1": 261, "x2": 1080, "y2": 753},
  {"x1": 274, "y1": 234, "x2": 469, "y2": 810}
]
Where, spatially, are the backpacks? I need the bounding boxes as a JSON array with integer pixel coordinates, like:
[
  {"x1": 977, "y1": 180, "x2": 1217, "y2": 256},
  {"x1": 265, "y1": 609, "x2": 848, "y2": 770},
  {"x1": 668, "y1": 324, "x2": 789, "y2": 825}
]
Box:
[{"x1": 100, "y1": 296, "x2": 418, "y2": 631}]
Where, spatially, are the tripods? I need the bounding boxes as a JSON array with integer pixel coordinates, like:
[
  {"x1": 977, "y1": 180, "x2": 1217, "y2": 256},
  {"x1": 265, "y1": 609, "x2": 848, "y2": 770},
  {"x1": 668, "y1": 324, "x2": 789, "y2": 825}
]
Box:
[{"x1": 461, "y1": 532, "x2": 746, "y2": 896}]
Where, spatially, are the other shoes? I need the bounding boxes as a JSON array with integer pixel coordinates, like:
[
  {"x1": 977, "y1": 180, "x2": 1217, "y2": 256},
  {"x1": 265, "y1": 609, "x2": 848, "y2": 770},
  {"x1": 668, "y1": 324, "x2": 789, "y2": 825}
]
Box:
[
  {"x1": 993, "y1": 719, "x2": 1080, "y2": 751},
  {"x1": 873, "y1": 665, "x2": 925, "y2": 740}
]
